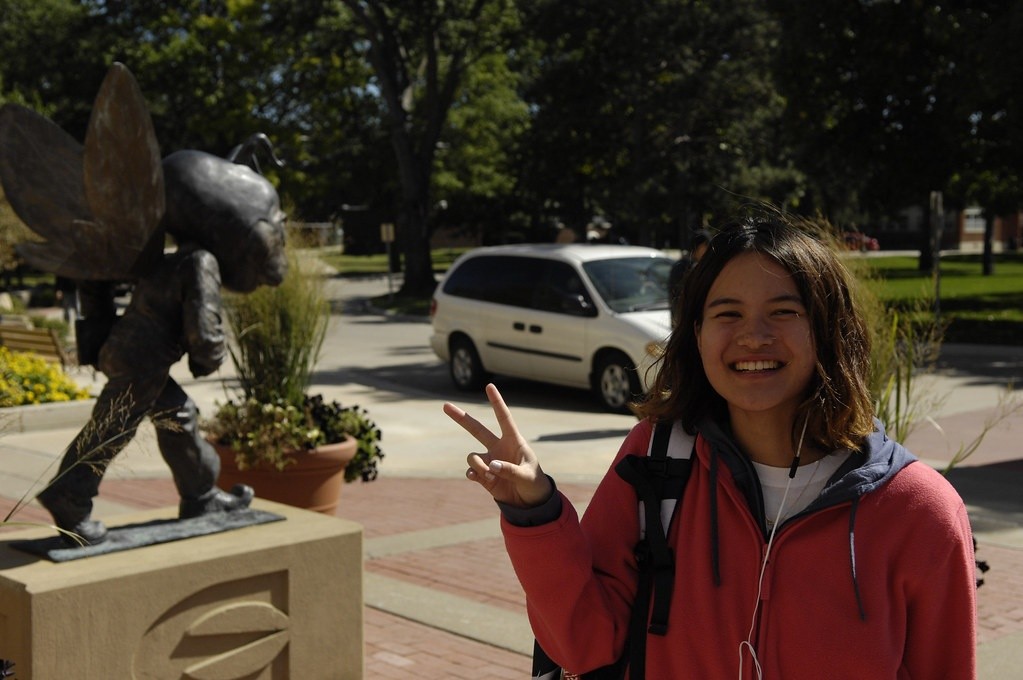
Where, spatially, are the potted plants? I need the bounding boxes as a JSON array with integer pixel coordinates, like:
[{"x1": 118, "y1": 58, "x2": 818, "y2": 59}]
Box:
[{"x1": 196, "y1": 217, "x2": 359, "y2": 517}]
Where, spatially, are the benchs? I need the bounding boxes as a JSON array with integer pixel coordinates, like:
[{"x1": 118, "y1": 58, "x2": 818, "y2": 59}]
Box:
[{"x1": 0, "y1": 324, "x2": 90, "y2": 374}]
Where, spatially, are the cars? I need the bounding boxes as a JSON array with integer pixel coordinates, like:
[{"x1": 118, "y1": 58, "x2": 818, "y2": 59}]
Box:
[{"x1": 428, "y1": 242, "x2": 679, "y2": 418}]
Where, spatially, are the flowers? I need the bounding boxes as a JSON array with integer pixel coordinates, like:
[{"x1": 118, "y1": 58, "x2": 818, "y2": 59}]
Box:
[{"x1": 202, "y1": 393, "x2": 384, "y2": 484}]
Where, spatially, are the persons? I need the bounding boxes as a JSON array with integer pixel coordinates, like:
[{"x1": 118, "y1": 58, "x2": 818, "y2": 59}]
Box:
[
  {"x1": 444, "y1": 216, "x2": 978, "y2": 680},
  {"x1": 667, "y1": 232, "x2": 710, "y2": 331},
  {"x1": 54, "y1": 276, "x2": 80, "y2": 325}
]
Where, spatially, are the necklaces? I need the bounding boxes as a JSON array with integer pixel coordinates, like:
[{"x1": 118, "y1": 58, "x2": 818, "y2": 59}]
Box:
[{"x1": 765, "y1": 451, "x2": 822, "y2": 527}]
